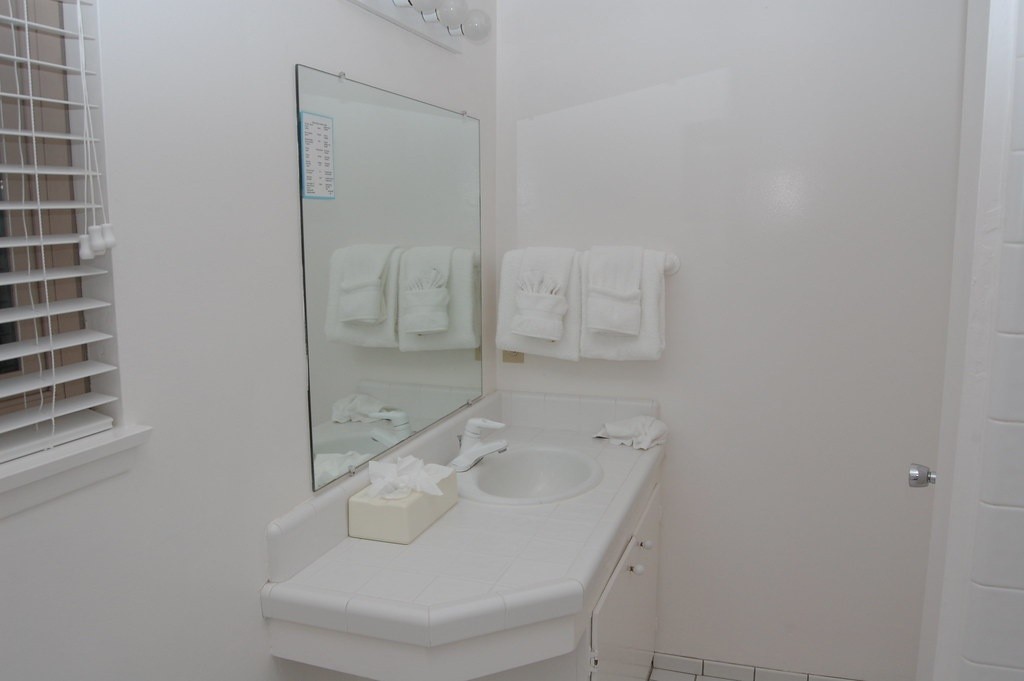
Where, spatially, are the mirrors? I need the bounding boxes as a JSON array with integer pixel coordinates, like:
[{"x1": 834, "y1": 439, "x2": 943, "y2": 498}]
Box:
[{"x1": 296, "y1": 63, "x2": 486, "y2": 493}]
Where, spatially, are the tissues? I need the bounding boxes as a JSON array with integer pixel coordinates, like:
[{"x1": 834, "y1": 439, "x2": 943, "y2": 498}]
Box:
[{"x1": 347, "y1": 455, "x2": 461, "y2": 548}]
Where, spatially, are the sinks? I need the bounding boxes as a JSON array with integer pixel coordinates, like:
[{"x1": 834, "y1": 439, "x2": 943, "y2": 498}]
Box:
[{"x1": 467, "y1": 440, "x2": 611, "y2": 507}]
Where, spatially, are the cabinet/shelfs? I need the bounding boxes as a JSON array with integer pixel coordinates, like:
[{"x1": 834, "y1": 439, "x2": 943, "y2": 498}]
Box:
[{"x1": 590, "y1": 482, "x2": 660, "y2": 681}]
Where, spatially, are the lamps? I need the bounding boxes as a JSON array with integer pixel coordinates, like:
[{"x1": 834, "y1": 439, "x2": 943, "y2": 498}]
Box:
[{"x1": 352, "y1": 0, "x2": 493, "y2": 56}]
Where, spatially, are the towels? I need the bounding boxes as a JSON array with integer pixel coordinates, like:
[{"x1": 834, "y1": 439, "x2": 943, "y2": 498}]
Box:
[
  {"x1": 399, "y1": 246, "x2": 486, "y2": 352},
  {"x1": 511, "y1": 243, "x2": 573, "y2": 343},
  {"x1": 493, "y1": 247, "x2": 586, "y2": 364},
  {"x1": 398, "y1": 243, "x2": 457, "y2": 337},
  {"x1": 585, "y1": 238, "x2": 646, "y2": 341},
  {"x1": 591, "y1": 410, "x2": 671, "y2": 452},
  {"x1": 328, "y1": 393, "x2": 396, "y2": 428},
  {"x1": 337, "y1": 243, "x2": 398, "y2": 326},
  {"x1": 322, "y1": 240, "x2": 401, "y2": 349},
  {"x1": 579, "y1": 241, "x2": 675, "y2": 363}
]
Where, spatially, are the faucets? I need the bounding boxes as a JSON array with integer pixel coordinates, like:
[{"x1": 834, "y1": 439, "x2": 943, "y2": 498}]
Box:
[
  {"x1": 445, "y1": 415, "x2": 509, "y2": 474},
  {"x1": 365, "y1": 409, "x2": 414, "y2": 448}
]
